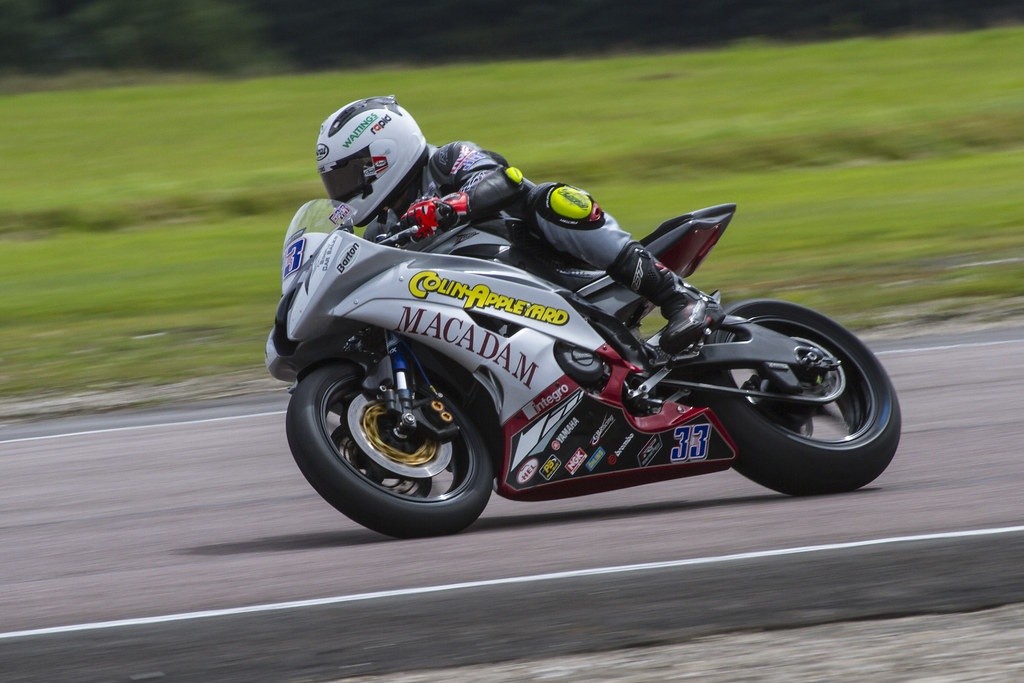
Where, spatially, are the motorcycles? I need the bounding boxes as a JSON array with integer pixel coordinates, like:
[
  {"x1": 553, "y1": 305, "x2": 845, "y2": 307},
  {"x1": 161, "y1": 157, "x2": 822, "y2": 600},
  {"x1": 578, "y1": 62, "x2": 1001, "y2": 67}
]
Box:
[{"x1": 263, "y1": 191, "x2": 901, "y2": 536}]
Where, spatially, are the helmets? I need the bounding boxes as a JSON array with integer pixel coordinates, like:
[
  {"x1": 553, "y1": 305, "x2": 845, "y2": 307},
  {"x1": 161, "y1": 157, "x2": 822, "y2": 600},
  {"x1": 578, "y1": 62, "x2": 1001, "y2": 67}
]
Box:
[{"x1": 317, "y1": 93, "x2": 425, "y2": 229}]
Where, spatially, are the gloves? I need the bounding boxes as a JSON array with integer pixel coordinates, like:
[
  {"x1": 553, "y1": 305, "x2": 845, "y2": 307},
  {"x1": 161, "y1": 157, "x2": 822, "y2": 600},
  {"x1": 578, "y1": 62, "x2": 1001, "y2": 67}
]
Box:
[{"x1": 394, "y1": 189, "x2": 469, "y2": 238}]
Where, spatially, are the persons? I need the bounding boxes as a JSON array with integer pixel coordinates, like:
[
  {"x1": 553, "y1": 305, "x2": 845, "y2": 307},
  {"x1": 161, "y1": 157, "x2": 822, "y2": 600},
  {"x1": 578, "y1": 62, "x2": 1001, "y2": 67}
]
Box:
[{"x1": 315, "y1": 93, "x2": 727, "y2": 354}]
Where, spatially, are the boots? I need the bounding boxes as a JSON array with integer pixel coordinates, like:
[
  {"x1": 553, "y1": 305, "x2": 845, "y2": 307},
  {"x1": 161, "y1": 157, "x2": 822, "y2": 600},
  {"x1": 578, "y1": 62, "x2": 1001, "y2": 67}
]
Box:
[{"x1": 606, "y1": 239, "x2": 725, "y2": 352}]
